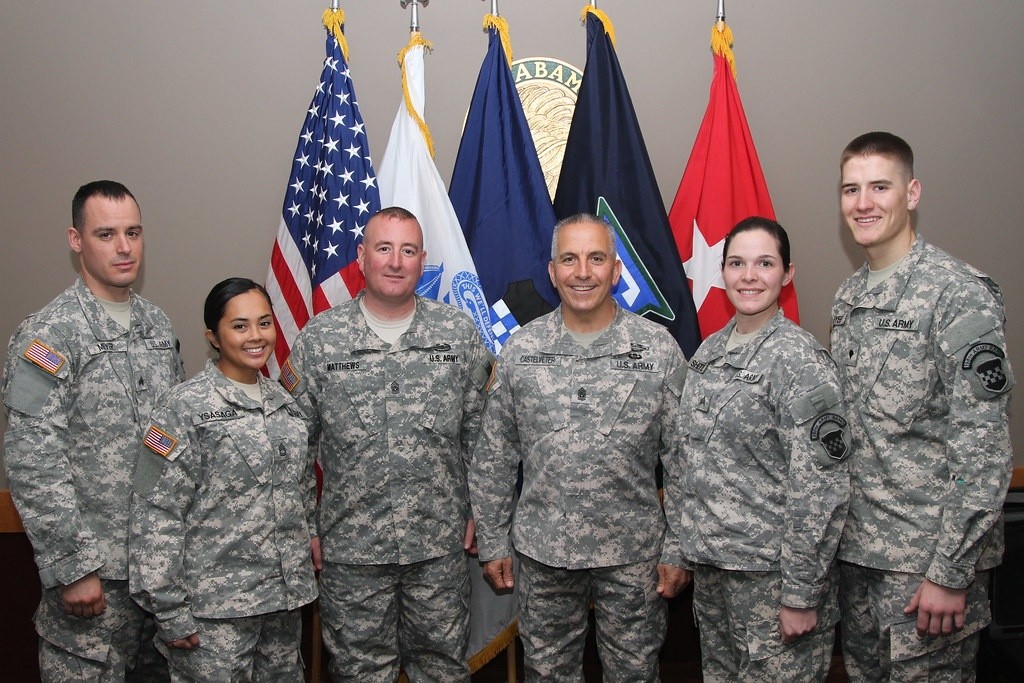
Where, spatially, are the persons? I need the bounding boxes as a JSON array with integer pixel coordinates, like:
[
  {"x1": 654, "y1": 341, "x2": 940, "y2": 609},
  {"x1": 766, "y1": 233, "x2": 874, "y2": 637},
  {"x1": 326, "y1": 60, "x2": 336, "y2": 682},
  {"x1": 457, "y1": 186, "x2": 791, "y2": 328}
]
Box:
[
  {"x1": 832, "y1": 131, "x2": 1015, "y2": 683},
  {"x1": 278, "y1": 207, "x2": 497, "y2": 683},
  {"x1": 677, "y1": 215, "x2": 850, "y2": 683},
  {"x1": 129, "y1": 277, "x2": 319, "y2": 683},
  {"x1": 465, "y1": 214, "x2": 691, "y2": 683},
  {"x1": 1, "y1": 179, "x2": 184, "y2": 682}
]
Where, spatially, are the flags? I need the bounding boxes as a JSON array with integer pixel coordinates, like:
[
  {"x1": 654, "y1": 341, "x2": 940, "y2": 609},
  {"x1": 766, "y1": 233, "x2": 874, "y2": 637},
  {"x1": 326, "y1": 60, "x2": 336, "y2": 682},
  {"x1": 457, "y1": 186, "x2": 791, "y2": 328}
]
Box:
[
  {"x1": 376, "y1": 32, "x2": 494, "y2": 354},
  {"x1": 447, "y1": 20, "x2": 561, "y2": 327},
  {"x1": 552, "y1": 10, "x2": 701, "y2": 362},
  {"x1": 669, "y1": 24, "x2": 800, "y2": 344},
  {"x1": 263, "y1": 10, "x2": 381, "y2": 384}
]
